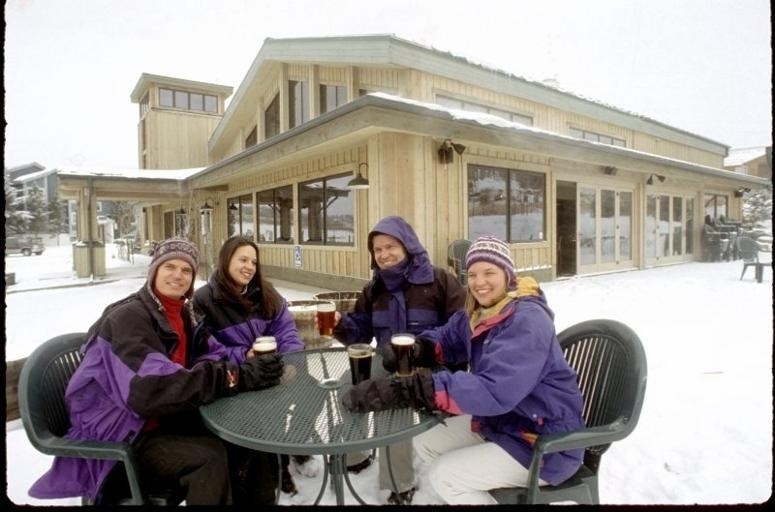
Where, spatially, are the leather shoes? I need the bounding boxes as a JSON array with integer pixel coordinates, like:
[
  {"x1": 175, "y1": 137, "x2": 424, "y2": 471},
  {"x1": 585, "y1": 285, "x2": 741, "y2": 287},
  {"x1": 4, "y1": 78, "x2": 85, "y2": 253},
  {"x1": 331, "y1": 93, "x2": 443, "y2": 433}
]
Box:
[{"x1": 387, "y1": 487, "x2": 415, "y2": 505}]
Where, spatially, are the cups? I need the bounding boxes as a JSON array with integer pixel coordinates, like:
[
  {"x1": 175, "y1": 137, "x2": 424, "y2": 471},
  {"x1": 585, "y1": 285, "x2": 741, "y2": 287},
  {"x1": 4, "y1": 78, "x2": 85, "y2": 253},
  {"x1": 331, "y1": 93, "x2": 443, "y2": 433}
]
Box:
[
  {"x1": 391, "y1": 332, "x2": 416, "y2": 373},
  {"x1": 253, "y1": 339, "x2": 277, "y2": 357},
  {"x1": 317, "y1": 299, "x2": 336, "y2": 338},
  {"x1": 349, "y1": 343, "x2": 374, "y2": 384},
  {"x1": 256, "y1": 336, "x2": 276, "y2": 342}
]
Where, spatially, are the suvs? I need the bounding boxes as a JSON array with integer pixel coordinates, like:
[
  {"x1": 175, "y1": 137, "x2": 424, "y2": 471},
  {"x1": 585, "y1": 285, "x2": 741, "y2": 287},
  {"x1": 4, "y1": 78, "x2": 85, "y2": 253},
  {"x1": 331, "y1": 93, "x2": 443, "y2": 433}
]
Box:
[{"x1": 5, "y1": 236, "x2": 45, "y2": 258}]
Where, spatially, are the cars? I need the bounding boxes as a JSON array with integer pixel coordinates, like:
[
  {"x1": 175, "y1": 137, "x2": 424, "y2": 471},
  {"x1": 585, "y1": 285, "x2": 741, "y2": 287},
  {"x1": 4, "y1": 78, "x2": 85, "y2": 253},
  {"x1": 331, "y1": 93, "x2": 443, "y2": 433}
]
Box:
[{"x1": 114, "y1": 234, "x2": 136, "y2": 246}]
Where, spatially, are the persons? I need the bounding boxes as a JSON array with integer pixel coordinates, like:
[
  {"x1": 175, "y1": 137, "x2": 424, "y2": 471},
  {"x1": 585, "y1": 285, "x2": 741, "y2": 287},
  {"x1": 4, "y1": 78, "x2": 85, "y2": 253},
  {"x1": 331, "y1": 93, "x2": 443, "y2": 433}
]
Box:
[
  {"x1": 23, "y1": 238, "x2": 283, "y2": 509},
  {"x1": 339, "y1": 236, "x2": 590, "y2": 506},
  {"x1": 192, "y1": 236, "x2": 329, "y2": 494},
  {"x1": 312, "y1": 214, "x2": 468, "y2": 506}
]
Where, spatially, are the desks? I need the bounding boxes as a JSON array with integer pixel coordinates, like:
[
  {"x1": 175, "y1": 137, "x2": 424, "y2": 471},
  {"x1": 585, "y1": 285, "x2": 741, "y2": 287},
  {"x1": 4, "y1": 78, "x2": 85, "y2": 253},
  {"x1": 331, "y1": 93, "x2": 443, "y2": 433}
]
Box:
[{"x1": 198, "y1": 346, "x2": 451, "y2": 506}]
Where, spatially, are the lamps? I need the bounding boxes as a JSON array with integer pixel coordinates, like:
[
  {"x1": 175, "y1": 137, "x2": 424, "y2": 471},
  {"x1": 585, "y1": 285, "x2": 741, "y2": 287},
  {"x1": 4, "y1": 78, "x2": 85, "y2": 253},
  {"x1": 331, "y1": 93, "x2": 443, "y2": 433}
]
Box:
[
  {"x1": 735, "y1": 187, "x2": 751, "y2": 197},
  {"x1": 201, "y1": 196, "x2": 220, "y2": 211},
  {"x1": 439, "y1": 139, "x2": 465, "y2": 163},
  {"x1": 647, "y1": 174, "x2": 665, "y2": 185},
  {"x1": 348, "y1": 163, "x2": 369, "y2": 187}
]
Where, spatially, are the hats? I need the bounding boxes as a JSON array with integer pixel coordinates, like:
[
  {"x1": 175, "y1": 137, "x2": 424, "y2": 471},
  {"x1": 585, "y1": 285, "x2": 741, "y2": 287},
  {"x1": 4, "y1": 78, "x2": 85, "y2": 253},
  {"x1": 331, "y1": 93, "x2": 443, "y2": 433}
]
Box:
[
  {"x1": 465, "y1": 232, "x2": 514, "y2": 287},
  {"x1": 147, "y1": 236, "x2": 201, "y2": 327}
]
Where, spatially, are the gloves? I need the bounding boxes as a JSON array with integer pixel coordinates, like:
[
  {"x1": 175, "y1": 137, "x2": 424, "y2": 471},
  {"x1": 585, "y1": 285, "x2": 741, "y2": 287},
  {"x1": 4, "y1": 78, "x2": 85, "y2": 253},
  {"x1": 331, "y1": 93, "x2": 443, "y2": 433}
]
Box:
[
  {"x1": 383, "y1": 341, "x2": 421, "y2": 369},
  {"x1": 342, "y1": 367, "x2": 436, "y2": 414},
  {"x1": 225, "y1": 355, "x2": 282, "y2": 393}
]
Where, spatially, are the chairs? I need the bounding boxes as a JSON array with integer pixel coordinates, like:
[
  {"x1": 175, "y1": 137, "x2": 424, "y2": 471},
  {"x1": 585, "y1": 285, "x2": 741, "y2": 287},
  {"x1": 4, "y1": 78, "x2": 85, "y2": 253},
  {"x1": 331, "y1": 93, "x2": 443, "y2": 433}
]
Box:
[
  {"x1": 447, "y1": 239, "x2": 473, "y2": 284},
  {"x1": 489, "y1": 319, "x2": 647, "y2": 505},
  {"x1": 737, "y1": 237, "x2": 772, "y2": 283},
  {"x1": 18, "y1": 336, "x2": 187, "y2": 506}
]
